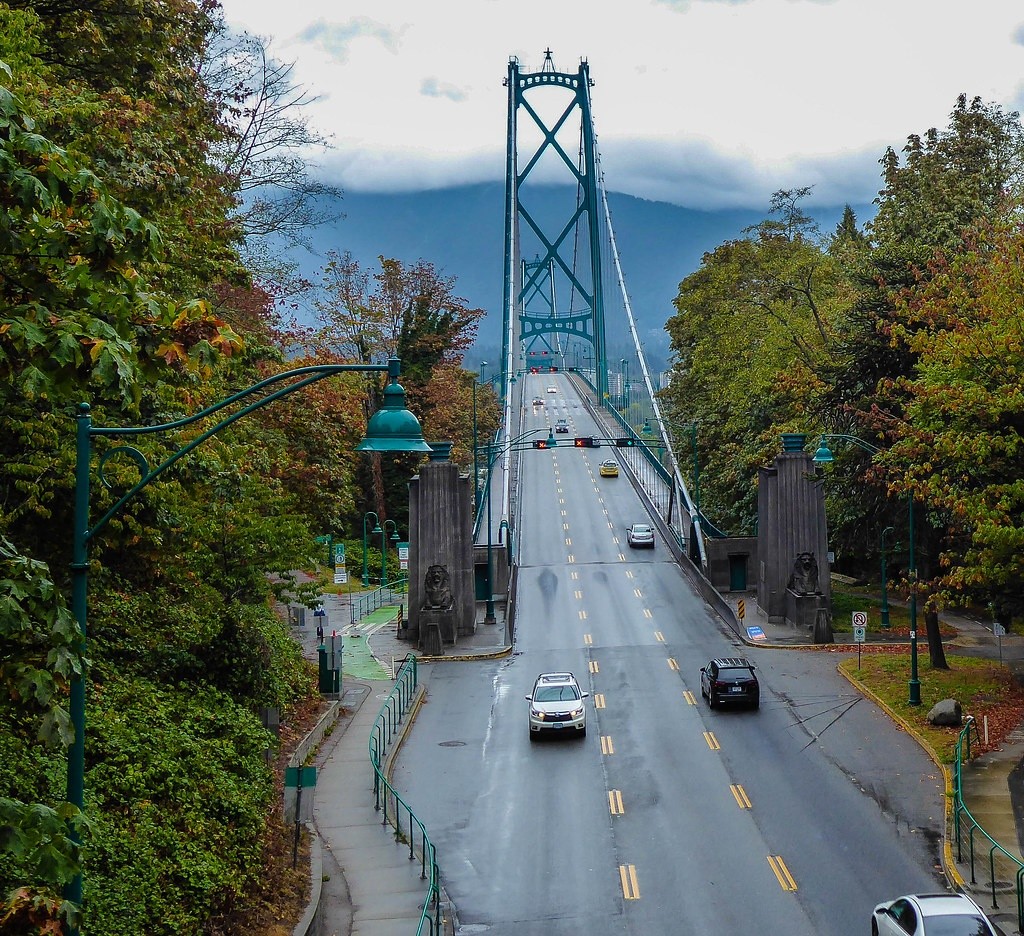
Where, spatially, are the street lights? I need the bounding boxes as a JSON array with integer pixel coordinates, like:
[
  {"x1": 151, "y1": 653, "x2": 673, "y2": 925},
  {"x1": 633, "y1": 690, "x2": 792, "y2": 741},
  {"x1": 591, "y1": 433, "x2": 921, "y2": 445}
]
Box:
[
  {"x1": 361, "y1": 511, "x2": 402, "y2": 590},
  {"x1": 471, "y1": 374, "x2": 560, "y2": 627},
  {"x1": 65, "y1": 355, "x2": 436, "y2": 907},
  {"x1": 811, "y1": 427, "x2": 925, "y2": 706}
]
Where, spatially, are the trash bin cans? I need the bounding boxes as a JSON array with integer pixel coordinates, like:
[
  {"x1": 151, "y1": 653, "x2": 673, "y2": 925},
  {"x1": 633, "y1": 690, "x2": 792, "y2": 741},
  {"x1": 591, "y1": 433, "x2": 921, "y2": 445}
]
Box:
[{"x1": 316, "y1": 645, "x2": 344, "y2": 700}]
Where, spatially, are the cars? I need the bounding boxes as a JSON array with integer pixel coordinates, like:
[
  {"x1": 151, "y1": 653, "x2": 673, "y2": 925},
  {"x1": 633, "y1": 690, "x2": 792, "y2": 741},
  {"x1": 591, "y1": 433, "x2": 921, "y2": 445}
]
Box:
[
  {"x1": 625, "y1": 523, "x2": 657, "y2": 549},
  {"x1": 598, "y1": 459, "x2": 620, "y2": 479},
  {"x1": 531, "y1": 396, "x2": 544, "y2": 406},
  {"x1": 547, "y1": 384, "x2": 557, "y2": 393},
  {"x1": 871, "y1": 892, "x2": 999, "y2": 936},
  {"x1": 588, "y1": 434, "x2": 601, "y2": 448}
]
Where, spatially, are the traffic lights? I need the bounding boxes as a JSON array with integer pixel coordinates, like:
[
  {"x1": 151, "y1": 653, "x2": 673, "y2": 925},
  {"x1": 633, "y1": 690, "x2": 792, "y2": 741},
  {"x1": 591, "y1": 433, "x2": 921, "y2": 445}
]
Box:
[
  {"x1": 533, "y1": 439, "x2": 552, "y2": 449},
  {"x1": 574, "y1": 437, "x2": 594, "y2": 447},
  {"x1": 529, "y1": 350, "x2": 580, "y2": 374},
  {"x1": 617, "y1": 438, "x2": 634, "y2": 448}
]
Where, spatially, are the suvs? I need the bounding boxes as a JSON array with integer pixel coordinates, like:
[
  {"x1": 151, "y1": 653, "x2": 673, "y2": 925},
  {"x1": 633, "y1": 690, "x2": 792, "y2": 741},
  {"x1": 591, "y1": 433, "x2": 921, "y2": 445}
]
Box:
[
  {"x1": 699, "y1": 657, "x2": 761, "y2": 714},
  {"x1": 525, "y1": 671, "x2": 590, "y2": 742}
]
